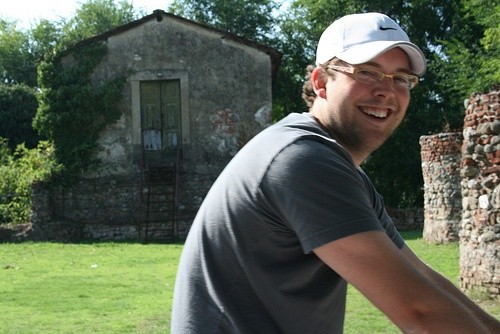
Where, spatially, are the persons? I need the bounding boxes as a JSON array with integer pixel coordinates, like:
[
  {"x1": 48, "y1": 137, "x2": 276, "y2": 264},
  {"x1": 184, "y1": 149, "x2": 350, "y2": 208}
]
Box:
[{"x1": 170, "y1": 11, "x2": 500, "y2": 334}]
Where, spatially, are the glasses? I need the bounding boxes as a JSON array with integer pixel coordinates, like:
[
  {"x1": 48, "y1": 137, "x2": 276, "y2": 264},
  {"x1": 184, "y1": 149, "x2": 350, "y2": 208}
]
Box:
[{"x1": 325, "y1": 65, "x2": 419, "y2": 90}]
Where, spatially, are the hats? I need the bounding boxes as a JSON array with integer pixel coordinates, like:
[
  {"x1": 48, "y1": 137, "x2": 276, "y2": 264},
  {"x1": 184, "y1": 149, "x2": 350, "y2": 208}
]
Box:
[{"x1": 316, "y1": 13, "x2": 426, "y2": 76}]
